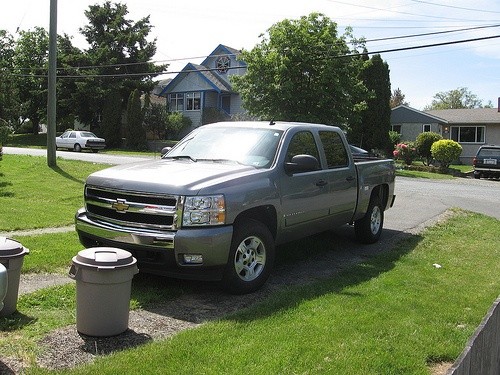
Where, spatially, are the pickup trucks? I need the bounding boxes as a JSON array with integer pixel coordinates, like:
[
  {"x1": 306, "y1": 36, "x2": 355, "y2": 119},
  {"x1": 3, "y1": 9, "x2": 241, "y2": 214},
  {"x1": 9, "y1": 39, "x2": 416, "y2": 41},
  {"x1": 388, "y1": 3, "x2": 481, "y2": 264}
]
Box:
[{"x1": 76, "y1": 118, "x2": 395, "y2": 292}]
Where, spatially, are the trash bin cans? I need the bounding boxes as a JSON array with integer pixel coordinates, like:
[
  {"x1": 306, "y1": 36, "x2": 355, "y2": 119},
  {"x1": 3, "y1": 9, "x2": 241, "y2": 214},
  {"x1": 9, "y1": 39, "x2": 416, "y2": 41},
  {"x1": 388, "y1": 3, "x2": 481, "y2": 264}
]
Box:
[
  {"x1": 0, "y1": 237, "x2": 30, "y2": 316},
  {"x1": 69, "y1": 246, "x2": 139, "y2": 336}
]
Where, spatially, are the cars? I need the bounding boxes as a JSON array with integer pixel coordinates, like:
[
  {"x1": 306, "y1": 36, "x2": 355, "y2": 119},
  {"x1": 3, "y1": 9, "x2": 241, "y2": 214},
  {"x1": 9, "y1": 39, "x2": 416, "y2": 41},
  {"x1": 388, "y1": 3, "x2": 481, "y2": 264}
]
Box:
[{"x1": 55, "y1": 130, "x2": 106, "y2": 152}]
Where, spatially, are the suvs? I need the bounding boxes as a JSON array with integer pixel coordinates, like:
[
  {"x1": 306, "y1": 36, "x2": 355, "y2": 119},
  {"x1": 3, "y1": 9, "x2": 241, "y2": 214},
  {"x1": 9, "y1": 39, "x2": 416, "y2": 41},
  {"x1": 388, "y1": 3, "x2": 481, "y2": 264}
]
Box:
[{"x1": 473, "y1": 144, "x2": 500, "y2": 182}]
